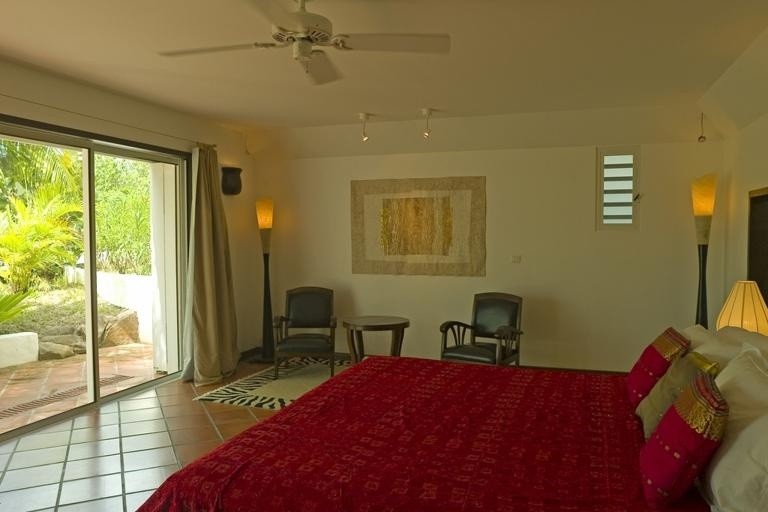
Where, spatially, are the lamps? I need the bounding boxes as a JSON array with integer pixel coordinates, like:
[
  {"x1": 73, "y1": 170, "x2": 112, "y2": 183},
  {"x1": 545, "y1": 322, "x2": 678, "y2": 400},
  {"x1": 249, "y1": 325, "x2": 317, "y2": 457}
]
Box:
[
  {"x1": 419, "y1": 108, "x2": 435, "y2": 141},
  {"x1": 714, "y1": 278, "x2": 768, "y2": 336},
  {"x1": 686, "y1": 173, "x2": 720, "y2": 329},
  {"x1": 222, "y1": 165, "x2": 243, "y2": 195},
  {"x1": 355, "y1": 109, "x2": 373, "y2": 144},
  {"x1": 254, "y1": 195, "x2": 276, "y2": 363}
]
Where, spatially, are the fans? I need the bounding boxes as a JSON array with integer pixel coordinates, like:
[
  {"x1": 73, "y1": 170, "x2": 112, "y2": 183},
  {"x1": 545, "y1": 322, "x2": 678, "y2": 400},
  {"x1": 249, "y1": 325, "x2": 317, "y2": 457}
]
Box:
[{"x1": 151, "y1": 0, "x2": 452, "y2": 85}]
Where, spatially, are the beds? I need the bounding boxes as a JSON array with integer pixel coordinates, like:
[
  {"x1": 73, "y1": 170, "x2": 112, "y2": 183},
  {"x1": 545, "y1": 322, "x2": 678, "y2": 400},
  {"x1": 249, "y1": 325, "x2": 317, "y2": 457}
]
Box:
[{"x1": 121, "y1": 324, "x2": 767, "y2": 512}]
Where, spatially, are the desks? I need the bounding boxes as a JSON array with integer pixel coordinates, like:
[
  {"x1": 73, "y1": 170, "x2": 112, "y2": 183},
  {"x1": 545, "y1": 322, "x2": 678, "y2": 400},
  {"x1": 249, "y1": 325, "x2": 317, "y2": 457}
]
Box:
[{"x1": 343, "y1": 315, "x2": 409, "y2": 365}]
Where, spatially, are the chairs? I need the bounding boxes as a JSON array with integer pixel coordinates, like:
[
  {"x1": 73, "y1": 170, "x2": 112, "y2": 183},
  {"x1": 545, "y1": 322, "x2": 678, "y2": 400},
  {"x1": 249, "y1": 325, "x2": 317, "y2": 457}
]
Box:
[
  {"x1": 438, "y1": 292, "x2": 522, "y2": 367},
  {"x1": 274, "y1": 284, "x2": 337, "y2": 378}
]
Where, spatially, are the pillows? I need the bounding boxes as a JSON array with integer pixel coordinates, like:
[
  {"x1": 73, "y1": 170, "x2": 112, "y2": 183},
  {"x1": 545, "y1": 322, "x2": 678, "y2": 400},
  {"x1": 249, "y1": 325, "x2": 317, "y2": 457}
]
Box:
[{"x1": 622, "y1": 325, "x2": 767, "y2": 512}]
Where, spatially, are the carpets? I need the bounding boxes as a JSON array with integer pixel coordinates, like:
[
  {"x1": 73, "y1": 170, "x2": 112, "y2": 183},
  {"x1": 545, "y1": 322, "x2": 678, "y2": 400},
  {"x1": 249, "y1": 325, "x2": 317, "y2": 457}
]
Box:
[{"x1": 187, "y1": 357, "x2": 356, "y2": 412}]
